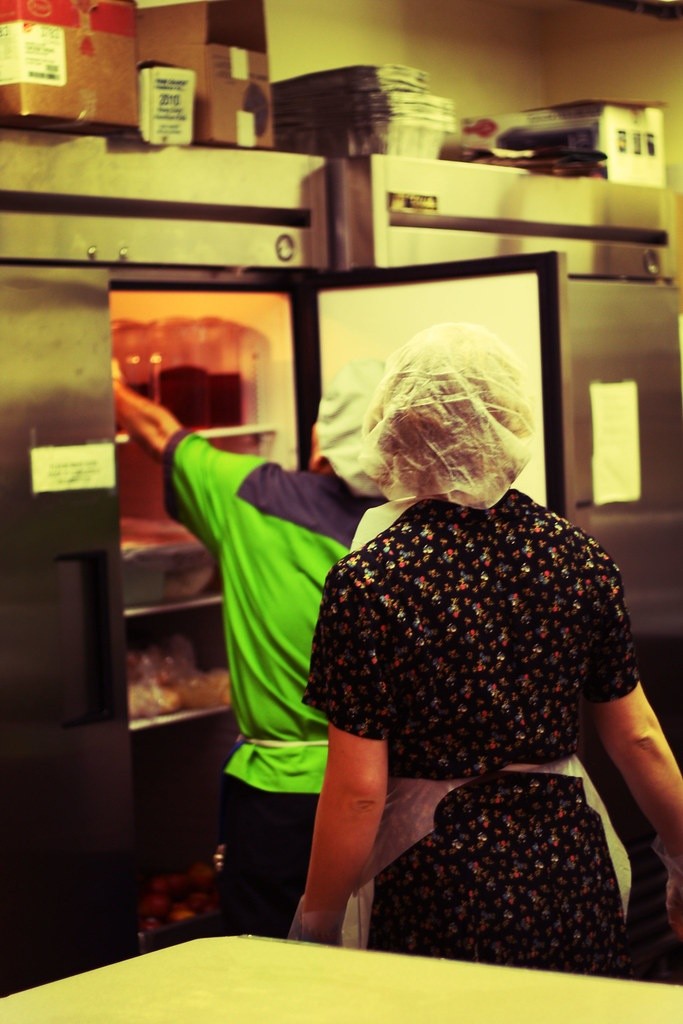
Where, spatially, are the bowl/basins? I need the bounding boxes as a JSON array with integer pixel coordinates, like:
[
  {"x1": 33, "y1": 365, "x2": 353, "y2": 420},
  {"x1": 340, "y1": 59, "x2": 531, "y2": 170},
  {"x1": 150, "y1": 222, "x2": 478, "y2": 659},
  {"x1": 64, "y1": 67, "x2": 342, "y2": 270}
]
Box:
[{"x1": 120, "y1": 546, "x2": 217, "y2": 608}]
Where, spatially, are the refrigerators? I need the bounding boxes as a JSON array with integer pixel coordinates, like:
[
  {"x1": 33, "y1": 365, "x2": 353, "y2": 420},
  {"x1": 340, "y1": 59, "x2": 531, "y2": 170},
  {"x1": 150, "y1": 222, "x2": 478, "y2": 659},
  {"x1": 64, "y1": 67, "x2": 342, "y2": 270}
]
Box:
[{"x1": 0, "y1": 209, "x2": 572, "y2": 1005}]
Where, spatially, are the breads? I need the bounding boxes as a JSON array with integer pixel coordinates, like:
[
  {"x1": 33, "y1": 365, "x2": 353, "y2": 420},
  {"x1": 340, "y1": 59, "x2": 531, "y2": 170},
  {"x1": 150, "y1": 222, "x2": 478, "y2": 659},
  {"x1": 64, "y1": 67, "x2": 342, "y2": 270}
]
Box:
[
  {"x1": 137, "y1": 858, "x2": 217, "y2": 931},
  {"x1": 127, "y1": 634, "x2": 235, "y2": 719}
]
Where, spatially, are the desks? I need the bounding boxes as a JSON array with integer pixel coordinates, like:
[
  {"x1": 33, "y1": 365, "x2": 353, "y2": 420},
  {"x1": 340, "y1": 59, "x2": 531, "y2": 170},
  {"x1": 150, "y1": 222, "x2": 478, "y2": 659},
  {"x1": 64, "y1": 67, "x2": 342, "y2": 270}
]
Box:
[{"x1": 1, "y1": 933, "x2": 683, "y2": 1023}]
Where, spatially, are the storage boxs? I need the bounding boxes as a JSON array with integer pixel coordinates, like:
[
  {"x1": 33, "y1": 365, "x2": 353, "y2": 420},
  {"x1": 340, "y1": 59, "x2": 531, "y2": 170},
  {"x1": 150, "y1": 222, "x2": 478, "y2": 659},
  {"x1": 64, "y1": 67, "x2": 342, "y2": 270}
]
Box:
[
  {"x1": 0, "y1": 0, "x2": 278, "y2": 146},
  {"x1": 515, "y1": 102, "x2": 665, "y2": 188}
]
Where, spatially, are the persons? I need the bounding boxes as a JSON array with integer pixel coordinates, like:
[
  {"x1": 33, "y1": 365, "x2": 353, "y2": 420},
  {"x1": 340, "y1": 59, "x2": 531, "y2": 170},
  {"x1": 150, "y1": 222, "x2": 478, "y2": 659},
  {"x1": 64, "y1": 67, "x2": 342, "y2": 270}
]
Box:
[
  {"x1": 285, "y1": 321, "x2": 682, "y2": 977},
  {"x1": 108, "y1": 355, "x2": 390, "y2": 939}
]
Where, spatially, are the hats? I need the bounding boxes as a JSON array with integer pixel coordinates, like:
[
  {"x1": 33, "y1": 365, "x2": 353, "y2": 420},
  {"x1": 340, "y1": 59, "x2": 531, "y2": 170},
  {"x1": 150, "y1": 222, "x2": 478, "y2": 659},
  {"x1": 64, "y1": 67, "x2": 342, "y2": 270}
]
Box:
[{"x1": 320, "y1": 357, "x2": 384, "y2": 495}]
